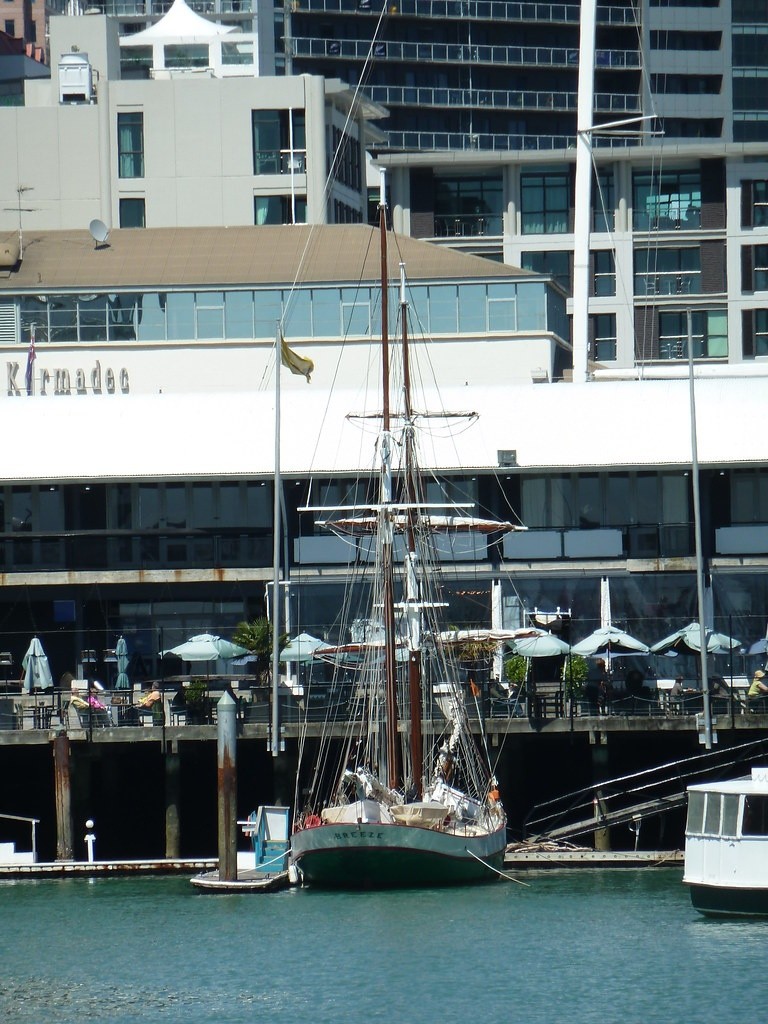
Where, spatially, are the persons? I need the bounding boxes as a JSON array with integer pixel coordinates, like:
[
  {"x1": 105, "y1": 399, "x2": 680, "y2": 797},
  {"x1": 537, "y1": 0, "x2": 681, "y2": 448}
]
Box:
[
  {"x1": 707, "y1": 678, "x2": 728, "y2": 694},
  {"x1": 172, "y1": 686, "x2": 200, "y2": 725},
  {"x1": 87, "y1": 687, "x2": 110, "y2": 727},
  {"x1": 70, "y1": 688, "x2": 97, "y2": 727},
  {"x1": 595, "y1": 658, "x2": 607, "y2": 684},
  {"x1": 748, "y1": 670, "x2": 768, "y2": 714},
  {"x1": 489, "y1": 679, "x2": 526, "y2": 717},
  {"x1": 126, "y1": 682, "x2": 161, "y2": 726},
  {"x1": 670, "y1": 675, "x2": 693, "y2": 714}
]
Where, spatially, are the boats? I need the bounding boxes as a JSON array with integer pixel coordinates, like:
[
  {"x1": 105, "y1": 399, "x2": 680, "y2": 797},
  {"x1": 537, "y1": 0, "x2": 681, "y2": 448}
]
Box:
[{"x1": 682, "y1": 768, "x2": 768, "y2": 918}]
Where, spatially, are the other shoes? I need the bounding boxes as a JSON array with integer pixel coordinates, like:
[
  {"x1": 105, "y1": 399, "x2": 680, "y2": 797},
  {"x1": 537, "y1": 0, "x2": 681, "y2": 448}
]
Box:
[
  {"x1": 518, "y1": 714, "x2": 527, "y2": 718},
  {"x1": 512, "y1": 714, "x2": 517, "y2": 718}
]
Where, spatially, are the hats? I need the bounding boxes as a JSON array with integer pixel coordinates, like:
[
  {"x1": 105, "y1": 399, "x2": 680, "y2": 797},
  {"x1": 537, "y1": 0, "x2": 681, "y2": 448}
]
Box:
[
  {"x1": 755, "y1": 670, "x2": 765, "y2": 678},
  {"x1": 675, "y1": 676, "x2": 683, "y2": 680}
]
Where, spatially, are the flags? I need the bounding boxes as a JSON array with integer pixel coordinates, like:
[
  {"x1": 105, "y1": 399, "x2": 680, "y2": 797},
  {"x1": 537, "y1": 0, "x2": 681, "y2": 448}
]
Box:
[
  {"x1": 24, "y1": 337, "x2": 37, "y2": 394},
  {"x1": 281, "y1": 337, "x2": 314, "y2": 384}
]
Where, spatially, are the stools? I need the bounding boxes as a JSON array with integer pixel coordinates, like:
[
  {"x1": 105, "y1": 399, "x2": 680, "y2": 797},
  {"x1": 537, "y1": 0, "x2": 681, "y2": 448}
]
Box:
[{"x1": 744, "y1": 689, "x2": 765, "y2": 714}]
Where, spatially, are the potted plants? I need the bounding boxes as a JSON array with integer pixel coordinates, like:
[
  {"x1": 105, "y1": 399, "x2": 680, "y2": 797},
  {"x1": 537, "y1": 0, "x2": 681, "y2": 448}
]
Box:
[
  {"x1": 185, "y1": 679, "x2": 207, "y2": 725},
  {"x1": 561, "y1": 655, "x2": 589, "y2": 716},
  {"x1": 151, "y1": 703, "x2": 163, "y2": 726}
]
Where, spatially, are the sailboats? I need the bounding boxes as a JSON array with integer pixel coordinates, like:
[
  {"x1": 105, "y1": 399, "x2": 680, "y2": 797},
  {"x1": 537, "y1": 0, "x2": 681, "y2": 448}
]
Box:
[{"x1": 287, "y1": 168, "x2": 509, "y2": 890}]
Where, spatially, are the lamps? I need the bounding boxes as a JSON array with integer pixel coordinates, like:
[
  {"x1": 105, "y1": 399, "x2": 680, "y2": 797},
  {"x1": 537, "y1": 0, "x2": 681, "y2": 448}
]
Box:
[{"x1": 498, "y1": 450, "x2": 519, "y2": 467}]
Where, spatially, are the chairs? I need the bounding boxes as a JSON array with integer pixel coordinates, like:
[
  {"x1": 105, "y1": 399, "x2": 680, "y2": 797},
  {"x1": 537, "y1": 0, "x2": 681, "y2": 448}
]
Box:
[
  {"x1": 709, "y1": 688, "x2": 732, "y2": 715},
  {"x1": 139, "y1": 698, "x2": 161, "y2": 726},
  {"x1": 72, "y1": 703, "x2": 103, "y2": 728},
  {"x1": 539, "y1": 691, "x2": 564, "y2": 718},
  {"x1": 168, "y1": 699, "x2": 192, "y2": 726},
  {"x1": 489, "y1": 695, "x2": 515, "y2": 718},
  {"x1": 662, "y1": 690, "x2": 684, "y2": 716},
  {"x1": 14, "y1": 700, "x2": 70, "y2": 730}
]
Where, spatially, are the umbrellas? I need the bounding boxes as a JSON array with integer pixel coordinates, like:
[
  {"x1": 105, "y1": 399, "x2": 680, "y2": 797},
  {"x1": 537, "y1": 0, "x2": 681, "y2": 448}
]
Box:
[
  {"x1": 231, "y1": 630, "x2": 364, "y2": 722},
  {"x1": 114, "y1": 636, "x2": 130, "y2": 689},
  {"x1": 505, "y1": 627, "x2": 572, "y2": 718},
  {"x1": 734, "y1": 638, "x2": 768, "y2": 655},
  {"x1": 570, "y1": 621, "x2": 650, "y2": 715},
  {"x1": 649, "y1": 619, "x2": 742, "y2": 686},
  {"x1": 364, "y1": 646, "x2": 455, "y2": 663},
  {"x1": 22, "y1": 637, "x2": 54, "y2": 729},
  {"x1": 156, "y1": 630, "x2": 257, "y2": 725}
]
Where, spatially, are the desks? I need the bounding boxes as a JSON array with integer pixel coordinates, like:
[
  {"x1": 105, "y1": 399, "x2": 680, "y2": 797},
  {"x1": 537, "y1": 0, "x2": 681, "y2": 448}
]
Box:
[
  {"x1": 24, "y1": 705, "x2": 59, "y2": 729},
  {"x1": 105, "y1": 704, "x2": 132, "y2": 727},
  {"x1": 683, "y1": 691, "x2": 703, "y2": 715},
  {"x1": 517, "y1": 695, "x2": 546, "y2": 718}
]
